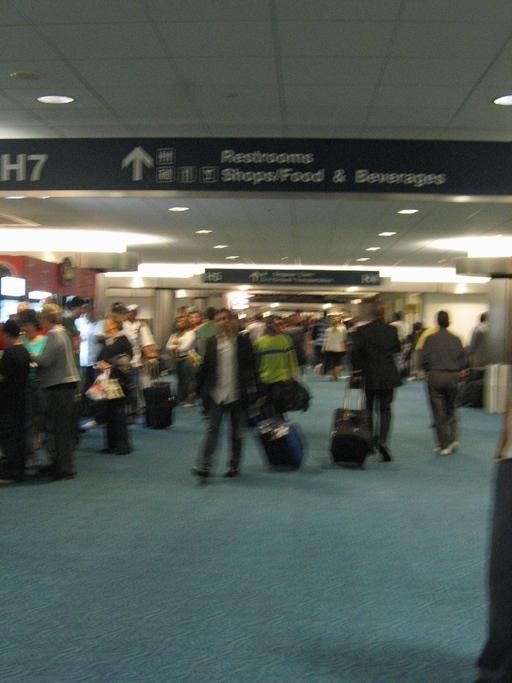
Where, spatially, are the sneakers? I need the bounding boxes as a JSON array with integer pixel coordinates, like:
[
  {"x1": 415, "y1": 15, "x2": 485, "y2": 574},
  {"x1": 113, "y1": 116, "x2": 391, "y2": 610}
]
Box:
[
  {"x1": 190, "y1": 466, "x2": 242, "y2": 481},
  {"x1": 378, "y1": 439, "x2": 459, "y2": 461},
  {"x1": 80, "y1": 419, "x2": 107, "y2": 431},
  {"x1": 96, "y1": 443, "x2": 132, "y2": 455},
  {"x1": 0, "y1": 460, "x2": 77, "y2": 485}
]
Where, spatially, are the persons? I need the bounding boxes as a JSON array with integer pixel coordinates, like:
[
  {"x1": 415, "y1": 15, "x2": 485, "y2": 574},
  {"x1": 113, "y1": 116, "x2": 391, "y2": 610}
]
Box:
[
  {"x1": 162, "y1": 305, "x2": 300, "y2": 483},
  {"x1": 1, "y1": 294, "x2": 159, "y2": 485},
  {"x1": 292, "y1": 296, "x2": 488, "y2": 462}
]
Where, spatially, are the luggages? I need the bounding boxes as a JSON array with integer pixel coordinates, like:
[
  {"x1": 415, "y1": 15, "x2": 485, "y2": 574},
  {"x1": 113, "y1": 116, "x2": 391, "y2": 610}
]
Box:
[
  {"x1": 329, "y1": 376, "x2": 373, "y2": 468},
  {"x1": 256, "y1": 417, "x2": 305, "y2": 472},
  {"x1": 141, "y1": 384, "x2": 172, "y2": 430}
]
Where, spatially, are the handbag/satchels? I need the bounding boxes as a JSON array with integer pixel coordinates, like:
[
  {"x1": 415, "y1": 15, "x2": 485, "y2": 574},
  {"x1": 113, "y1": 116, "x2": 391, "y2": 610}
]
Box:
[
  {"x1": 175, "y1": 351, "x2": 189, "y2": 361},
  {"x1": 282, "y1": 380, "x2": 311, "y2": 412},
  {"x1": 186, "y1": 349, "x2": 202, "y2": 369}
]
